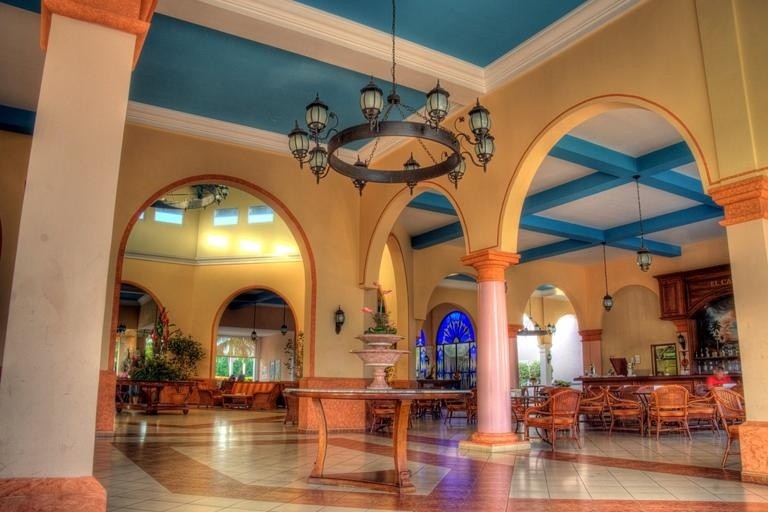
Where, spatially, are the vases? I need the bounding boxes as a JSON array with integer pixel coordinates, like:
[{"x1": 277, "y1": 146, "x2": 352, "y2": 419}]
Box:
[{"x1": 346, "y1": 335, "x2": 411, "y2": 392}]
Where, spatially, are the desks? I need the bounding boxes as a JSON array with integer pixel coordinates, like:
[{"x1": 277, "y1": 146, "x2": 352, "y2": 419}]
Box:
[
  {"x1": 116, "y1": 378, "x2": 203, "y2": 416},
  {"x1": 283, "y1": 385, "x2": 473, "y2": 494}
]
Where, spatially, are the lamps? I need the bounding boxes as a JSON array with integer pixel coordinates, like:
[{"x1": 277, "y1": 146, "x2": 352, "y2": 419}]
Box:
[
  {"x1": 335, "y1": 304, "x2": 345, "y2": 333},
  {"x1": 280, "y1": 302, "x2": 288, "y2": 335},
  {"x1": 633, "y1": 175, "x2": 652, "y2": 272},
  {"x1": 602, "y1": 242, "x2": 613, "y2": 311},
  {"x1": 514, "y1": 293, "x2": 558, "y2": 337},
  {"x1": 151, "y1": 182, "x2": 229, "y2": 213},
  {"x1": 288, "y1": 0, "x2": 494, "y2": 196},
  {"x1": 252, "y1": 302, "x2": 256, "y2": 339},
  {"x1": 624, "y1": 354, "x2": 642, "y2": 379}
]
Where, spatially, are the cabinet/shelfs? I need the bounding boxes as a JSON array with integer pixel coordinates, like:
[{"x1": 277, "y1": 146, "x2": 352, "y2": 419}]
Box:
[{"x1": 691, "y1": 354, "x2": 741, "y2": 377}]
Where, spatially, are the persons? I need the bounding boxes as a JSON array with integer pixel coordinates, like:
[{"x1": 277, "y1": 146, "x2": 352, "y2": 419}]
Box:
[{"x1": 703, "y1": 367, "x2": 734, "y2": 392}]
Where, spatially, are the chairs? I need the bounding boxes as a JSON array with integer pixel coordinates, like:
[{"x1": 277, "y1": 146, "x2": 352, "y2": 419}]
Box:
[
  {"x1": 364, "y1": 385, "x2": 531, "y2": 435},
  {"x1": 524, "y1": 385, "x2": 744, "y2": 452},
  {"x1": 710, "y1": 386, "x2": 745, "y2": 466},
  {"x1": 197, "y1": 382, "x2": 282, "y2": 410}
]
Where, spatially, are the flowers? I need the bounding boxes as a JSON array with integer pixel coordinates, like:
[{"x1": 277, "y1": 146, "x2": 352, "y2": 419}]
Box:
[{"x1": 351, "y1": 279, "x2": 409, "y2": 335}]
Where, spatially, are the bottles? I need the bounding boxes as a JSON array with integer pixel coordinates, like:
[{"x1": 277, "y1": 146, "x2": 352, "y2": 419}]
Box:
[
  {"x1": 628, "y1": 362, "x2": 634, "y2": 376},
  {"x1": 694, "y1": 345, "x2": 740, "y2": 357},
  {"x1": 696, "y1": 360, "x2": 739, "y2": 374},
  {"x1": 590, "y1": 362, "x2": 595, "y2": 376}
]
